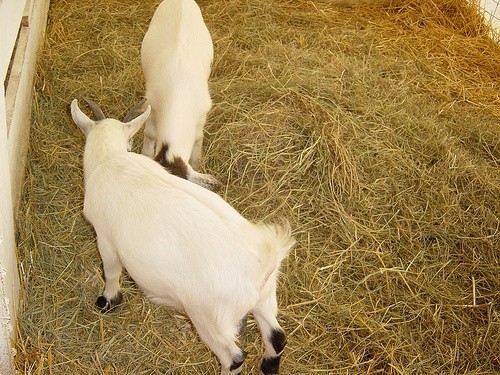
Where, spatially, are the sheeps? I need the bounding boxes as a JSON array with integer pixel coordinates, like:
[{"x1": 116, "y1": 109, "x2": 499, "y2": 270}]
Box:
[
  {"x1": 69, "y1": 95, "x2": 298, "y2": 375},
  {"x1": 139, "y1": 0, "x2": 227, "y2": 195}
]
